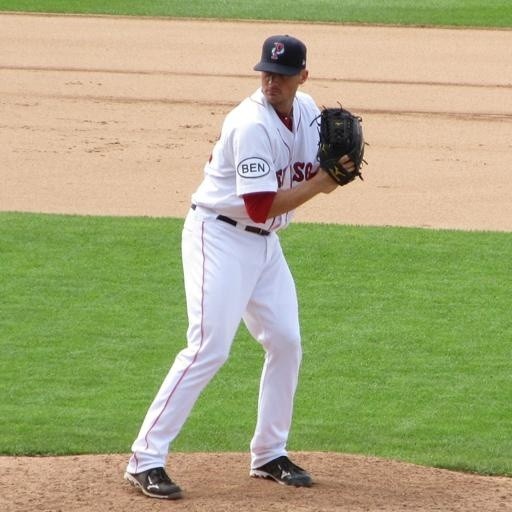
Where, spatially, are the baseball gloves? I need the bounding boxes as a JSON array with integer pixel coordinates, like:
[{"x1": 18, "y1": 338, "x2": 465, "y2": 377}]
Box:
[{"x1": 310, "y1": 101, "x2": 369, "y2": 186}]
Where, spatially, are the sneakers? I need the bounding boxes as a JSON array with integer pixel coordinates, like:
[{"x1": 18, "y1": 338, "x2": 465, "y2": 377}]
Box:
[
  {"x1": 250, "y1": 456, "x2": 311, "y2": 487},
  {"x1": 124, "y1": 468, "x2": 182, "y2": 499}
]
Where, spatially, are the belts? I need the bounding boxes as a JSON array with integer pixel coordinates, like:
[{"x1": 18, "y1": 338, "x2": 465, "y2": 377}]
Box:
[{"x1": 191, "y1": 203, "x2": 273, "y2": 235}]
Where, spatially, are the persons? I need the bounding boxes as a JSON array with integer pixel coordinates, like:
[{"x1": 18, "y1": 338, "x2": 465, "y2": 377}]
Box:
[{"x1": 122, "y1": 33, "x2": 370, "y2": 502}]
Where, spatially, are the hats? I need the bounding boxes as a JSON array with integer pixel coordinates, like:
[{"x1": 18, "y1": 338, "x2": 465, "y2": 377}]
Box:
[{"x1": 254, "y1": 35, "x2": 307, "y2": 76}]
